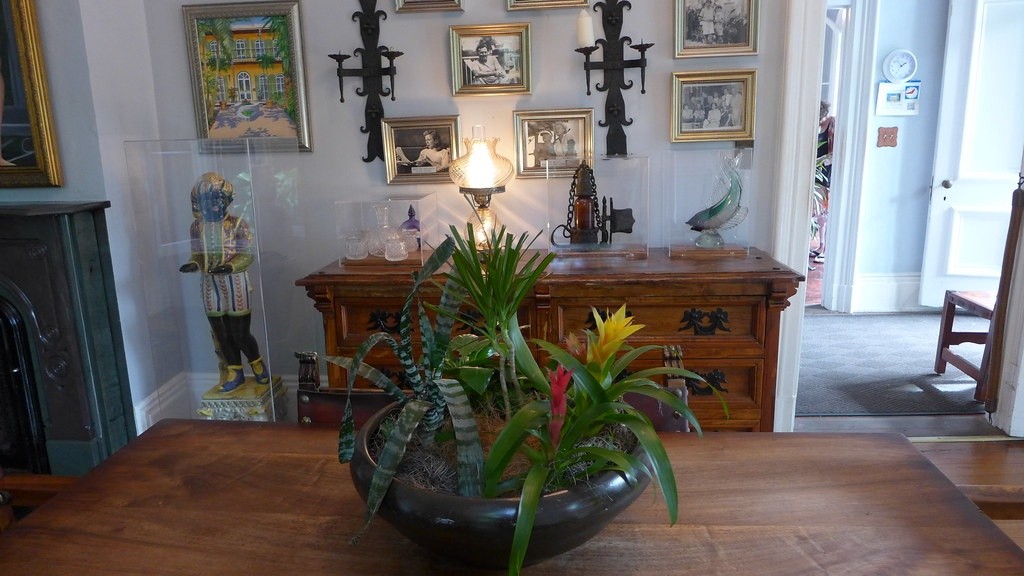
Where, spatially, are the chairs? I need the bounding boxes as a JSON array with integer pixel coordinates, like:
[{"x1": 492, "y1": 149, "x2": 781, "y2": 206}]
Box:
[{"x1": 935, "y1": 289, "x2": 1001, "y2": 406}]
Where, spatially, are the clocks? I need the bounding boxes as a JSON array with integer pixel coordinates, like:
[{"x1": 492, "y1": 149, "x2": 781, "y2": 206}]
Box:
[{"x1": 881, "y1": 50, "x2": 918, "y2": 84}]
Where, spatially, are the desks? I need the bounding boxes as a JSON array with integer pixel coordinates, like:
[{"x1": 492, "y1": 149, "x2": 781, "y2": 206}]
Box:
[{"x1": 0, "y1": 416, "x2": 1024, "y2": 576}]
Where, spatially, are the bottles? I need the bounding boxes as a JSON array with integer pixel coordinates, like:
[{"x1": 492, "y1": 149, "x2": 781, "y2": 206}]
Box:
[
  {"x1": 368, "y1": 204, "x2": 399, "y2": 258},
  {"x1": 398, "y1": 204, "x2": 428, "y2": 248}
]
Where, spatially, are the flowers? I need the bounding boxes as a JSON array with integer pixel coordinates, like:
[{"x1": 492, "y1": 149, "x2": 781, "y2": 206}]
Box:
[{"x1": 316, "y1": 215, "x2": 732, "y2": 576}]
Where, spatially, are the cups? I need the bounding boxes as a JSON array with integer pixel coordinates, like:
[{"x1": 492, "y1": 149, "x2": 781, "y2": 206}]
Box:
[{"x1": 343, "y1": 229, "x2": 419, "y2": 262}]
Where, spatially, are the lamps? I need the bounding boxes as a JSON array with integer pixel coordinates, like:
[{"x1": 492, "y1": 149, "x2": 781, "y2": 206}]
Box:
[
  {"x1": 670, "y1": 67, "x2": 758, "y2": 144},
  {"x1": 674, "y1": 0, "x2": 762, "y2": 59},
  {"x1": 448, "y1": 124, "x2": 515, "y2": 262}
]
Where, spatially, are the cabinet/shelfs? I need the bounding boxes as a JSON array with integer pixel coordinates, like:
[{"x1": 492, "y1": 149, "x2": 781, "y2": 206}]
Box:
[{"x1": 296, "y1": 246, "x2": 808, "y2": 433}]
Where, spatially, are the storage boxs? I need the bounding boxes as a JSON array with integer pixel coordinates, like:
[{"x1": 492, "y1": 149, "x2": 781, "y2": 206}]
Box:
[
  {"x1": 660, "y1": 148, "x2": 751, "y2": 260},
  {"x1": 125, "y1": 137, "x2": 307, "y2": 424},
  {"x1": 542, "y1": 154, "x2": 650, "y2": 261},
  {"x1": 336, "y1": 192, "x2": 440, "y2": 268}
]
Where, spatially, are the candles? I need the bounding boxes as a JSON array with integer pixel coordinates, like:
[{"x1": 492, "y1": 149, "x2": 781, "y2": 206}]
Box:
[{"x1": 577, "y1": 7, "x2": 595, "y2": 47}]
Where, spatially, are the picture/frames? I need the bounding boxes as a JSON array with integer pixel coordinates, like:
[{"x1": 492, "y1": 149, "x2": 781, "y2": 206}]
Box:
[
  {"x1": 505, "y1": 0, "x2": 590, "y2": 12},
  {"x1": 0, "y1": 0, "x2": 63, "y2": 189},
  {"x1": 394, "y1": 0, "x2": 466, "y2": 13},
  {"x1": 381, "y1": 114, "x2": 463, "y2": 186},
  {"x1": 449, "y1": 22, "x2": 533, "y2": 96},
  {"x1": 182, "y1": 0, "x2": 313, "y2": 155},
  {"x1": 512, "y1": 107, "x2": 595, "y2": 179}
]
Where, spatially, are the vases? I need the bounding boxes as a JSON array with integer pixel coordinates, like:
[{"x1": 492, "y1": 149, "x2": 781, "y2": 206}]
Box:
[{"x1": 345, "y1": 403, "x2": 653, "y2": 576}]
